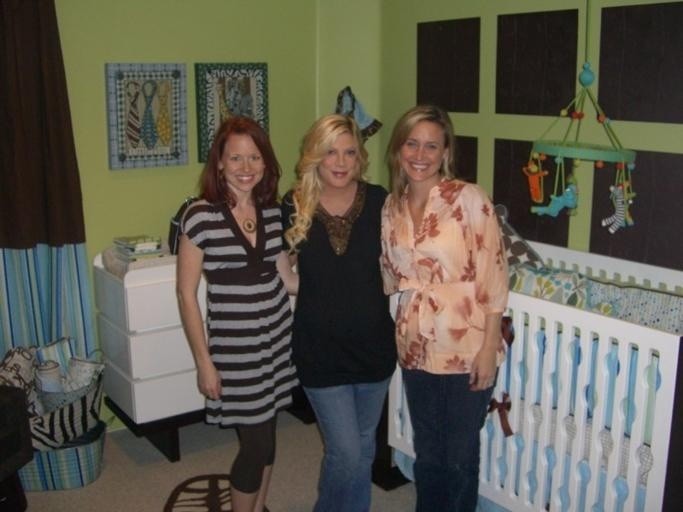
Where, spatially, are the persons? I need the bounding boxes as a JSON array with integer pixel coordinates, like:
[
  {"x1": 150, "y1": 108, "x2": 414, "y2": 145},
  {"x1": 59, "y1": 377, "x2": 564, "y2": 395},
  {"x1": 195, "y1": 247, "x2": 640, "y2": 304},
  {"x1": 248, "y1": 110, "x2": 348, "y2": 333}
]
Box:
[
  {"x1": 282, "y1": 113, "x2": 400, "y2": 511},
  {"x1": 378, "y1": 102, "x2": 510, "y2": 511},
  {"x1": 176, "y1": 114, "x2": 302, "y2": 510}
]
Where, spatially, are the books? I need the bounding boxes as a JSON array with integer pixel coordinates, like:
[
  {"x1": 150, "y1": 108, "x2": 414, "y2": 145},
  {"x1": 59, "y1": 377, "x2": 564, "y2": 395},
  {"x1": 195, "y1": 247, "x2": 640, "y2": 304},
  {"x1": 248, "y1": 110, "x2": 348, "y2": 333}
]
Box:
[{"x1": 113, "y1": 235, "x2": 165, "y2": 263}]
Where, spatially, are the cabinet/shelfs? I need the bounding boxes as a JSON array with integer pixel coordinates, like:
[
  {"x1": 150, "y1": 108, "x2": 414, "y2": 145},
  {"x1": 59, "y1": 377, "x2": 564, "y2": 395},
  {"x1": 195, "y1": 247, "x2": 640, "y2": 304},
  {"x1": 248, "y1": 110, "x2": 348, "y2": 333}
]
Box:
[{"x1": 90, "y1": 251, "x2": 319, "y2": 463}]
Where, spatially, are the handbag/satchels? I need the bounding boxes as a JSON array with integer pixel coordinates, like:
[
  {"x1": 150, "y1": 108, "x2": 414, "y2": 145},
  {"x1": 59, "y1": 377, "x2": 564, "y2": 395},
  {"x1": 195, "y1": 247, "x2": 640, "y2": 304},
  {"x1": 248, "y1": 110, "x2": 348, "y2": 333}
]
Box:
[{"x1": 28, "y1": 348, "x2": 108, "y2": 452}]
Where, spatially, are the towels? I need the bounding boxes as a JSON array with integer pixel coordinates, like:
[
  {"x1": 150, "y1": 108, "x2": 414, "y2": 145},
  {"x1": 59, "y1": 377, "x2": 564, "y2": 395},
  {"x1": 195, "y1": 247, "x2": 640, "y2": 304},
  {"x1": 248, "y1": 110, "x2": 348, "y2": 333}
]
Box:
[{"x1": 0, "y1": 336, "x2": 103, "y2": 391}]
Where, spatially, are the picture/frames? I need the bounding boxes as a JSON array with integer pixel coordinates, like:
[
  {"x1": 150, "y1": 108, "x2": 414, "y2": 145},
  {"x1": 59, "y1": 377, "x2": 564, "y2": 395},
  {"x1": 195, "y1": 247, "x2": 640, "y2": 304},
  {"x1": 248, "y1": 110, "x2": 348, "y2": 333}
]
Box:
[
  {"x1": 105, "y1": 62, "x2": 188, "y2": 170},
  {"x1": 195, "y1": 62, "x2": 270, "y2": 163}
]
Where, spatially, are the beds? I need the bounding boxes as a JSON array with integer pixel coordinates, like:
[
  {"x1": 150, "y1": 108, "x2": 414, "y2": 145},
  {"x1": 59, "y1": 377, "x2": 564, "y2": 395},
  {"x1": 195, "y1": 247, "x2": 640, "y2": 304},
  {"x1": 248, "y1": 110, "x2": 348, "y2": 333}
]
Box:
[{"x1": 385, "y1": 243, "x2": 683, "y2": 512}]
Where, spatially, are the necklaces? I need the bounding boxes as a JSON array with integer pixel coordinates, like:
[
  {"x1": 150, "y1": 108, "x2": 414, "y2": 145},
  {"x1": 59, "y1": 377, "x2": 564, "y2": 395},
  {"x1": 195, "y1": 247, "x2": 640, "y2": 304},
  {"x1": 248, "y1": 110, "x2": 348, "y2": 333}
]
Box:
[{"x1": 241, "y1": 217, "x2": 256, "y2": 232}]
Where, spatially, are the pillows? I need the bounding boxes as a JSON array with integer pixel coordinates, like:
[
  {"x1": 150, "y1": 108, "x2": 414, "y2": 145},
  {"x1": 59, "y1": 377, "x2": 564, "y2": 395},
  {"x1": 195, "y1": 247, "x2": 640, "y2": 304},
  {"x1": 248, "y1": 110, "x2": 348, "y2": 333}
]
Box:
[{"x1": 495, "y1": 202, "x2": 546, "y2": 270}]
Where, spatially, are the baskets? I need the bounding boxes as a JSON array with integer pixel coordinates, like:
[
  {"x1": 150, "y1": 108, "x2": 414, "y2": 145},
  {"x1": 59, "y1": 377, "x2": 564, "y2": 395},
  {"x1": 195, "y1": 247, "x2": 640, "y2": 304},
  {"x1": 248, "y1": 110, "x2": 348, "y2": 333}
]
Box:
[{"x1": 17, "y1": 415, "x2": 116, "y2": 493}]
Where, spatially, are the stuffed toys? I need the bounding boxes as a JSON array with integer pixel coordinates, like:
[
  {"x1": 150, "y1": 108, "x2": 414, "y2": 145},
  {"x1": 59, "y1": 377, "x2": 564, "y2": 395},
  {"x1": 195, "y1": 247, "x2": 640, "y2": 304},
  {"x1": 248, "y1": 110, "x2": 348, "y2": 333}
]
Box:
[{"x1": 519, "y1": 60, "x2": 640, "y2": 234}]
